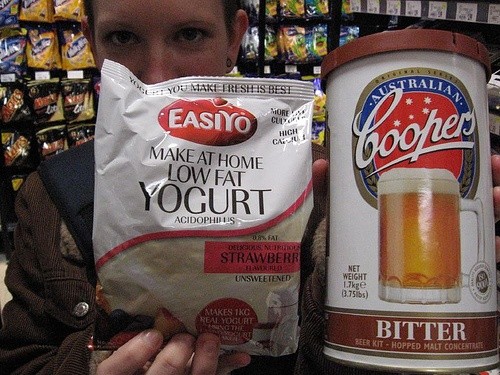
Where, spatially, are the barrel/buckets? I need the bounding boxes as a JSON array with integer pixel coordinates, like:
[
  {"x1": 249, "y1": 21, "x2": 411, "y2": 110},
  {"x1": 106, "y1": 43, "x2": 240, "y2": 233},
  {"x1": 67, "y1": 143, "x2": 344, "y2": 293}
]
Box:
[{"x1": 320, "y1": 30, "x2": 500, "y2": 374}]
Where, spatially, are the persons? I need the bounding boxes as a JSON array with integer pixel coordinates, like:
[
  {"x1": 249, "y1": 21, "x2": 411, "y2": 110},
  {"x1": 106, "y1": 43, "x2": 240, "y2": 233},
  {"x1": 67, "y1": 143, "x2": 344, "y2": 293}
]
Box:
[{"x1": 0, "y1": 0, "x2": 331, "y2": 375}]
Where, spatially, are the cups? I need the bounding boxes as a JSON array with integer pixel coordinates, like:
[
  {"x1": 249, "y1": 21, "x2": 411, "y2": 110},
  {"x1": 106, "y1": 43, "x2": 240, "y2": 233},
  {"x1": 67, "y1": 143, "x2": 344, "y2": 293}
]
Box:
[
  {"x1": 267, "y1": 302, "x2": 297, "y2": 356},
  {"x1": 376, "y1": 180, "x2": 484, "y2": 304}
]
